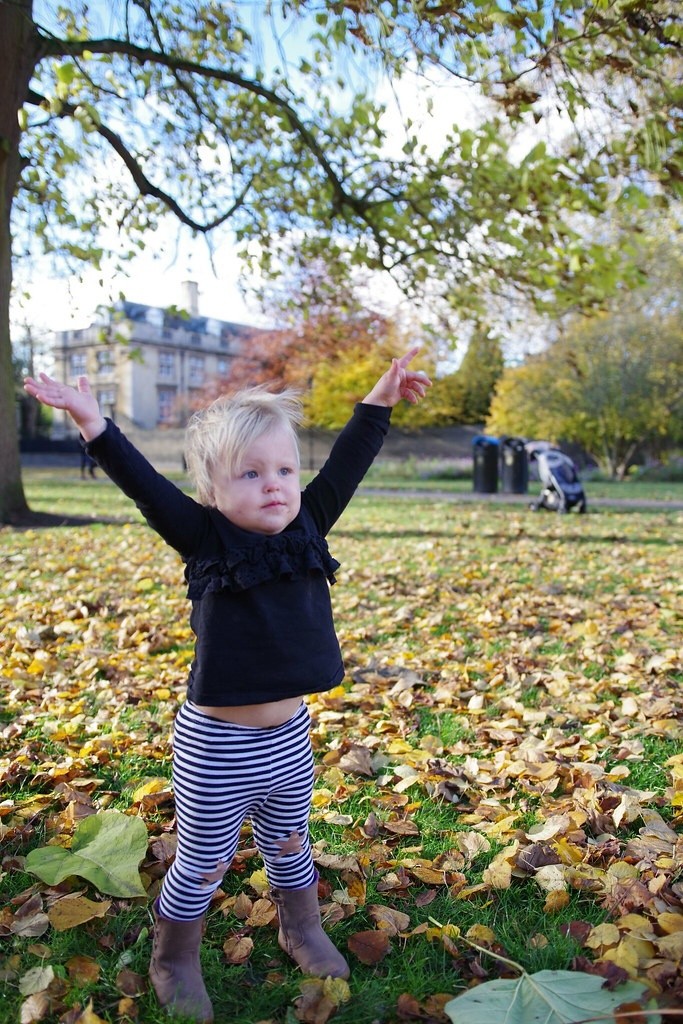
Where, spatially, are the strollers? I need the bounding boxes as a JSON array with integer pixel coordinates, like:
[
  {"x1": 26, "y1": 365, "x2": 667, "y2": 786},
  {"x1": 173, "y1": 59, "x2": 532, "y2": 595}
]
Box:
[{"x1": 523, "y1": 441, "x2": 588, "y2": 515}]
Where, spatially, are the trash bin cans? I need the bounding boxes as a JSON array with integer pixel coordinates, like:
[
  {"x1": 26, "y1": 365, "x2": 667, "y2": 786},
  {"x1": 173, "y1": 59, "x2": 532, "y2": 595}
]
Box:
[
  {"x1": 471, "y1": 435, "x2": 500, "y2": 493},
  {"x1": 502, "y1": 437, "x2": 528, "y2": 494}
]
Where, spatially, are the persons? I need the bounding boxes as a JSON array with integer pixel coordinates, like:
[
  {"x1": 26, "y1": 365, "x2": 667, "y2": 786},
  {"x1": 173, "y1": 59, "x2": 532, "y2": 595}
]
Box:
[
  {"x1": 23, "y1": 348, "x2": 434, "y2": 1024},
  {"x1": 77, "y1": 434, "x2": 99, "y2": 480}
]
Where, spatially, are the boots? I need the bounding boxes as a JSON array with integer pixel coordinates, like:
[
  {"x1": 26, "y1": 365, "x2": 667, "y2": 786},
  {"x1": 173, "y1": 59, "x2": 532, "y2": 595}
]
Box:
[
  {"x1": 269, "y1": 867, "x2": 350, "y2": 981},
  {"x1": 148, "y1": 895, "x2": 214, "y2": 1024}
]
